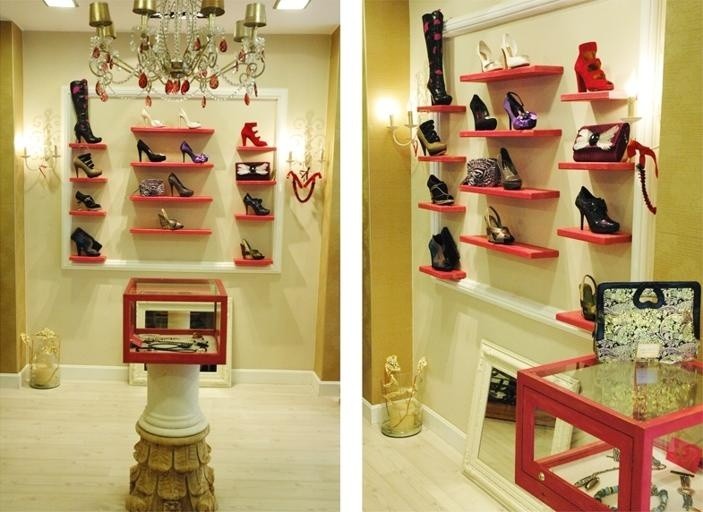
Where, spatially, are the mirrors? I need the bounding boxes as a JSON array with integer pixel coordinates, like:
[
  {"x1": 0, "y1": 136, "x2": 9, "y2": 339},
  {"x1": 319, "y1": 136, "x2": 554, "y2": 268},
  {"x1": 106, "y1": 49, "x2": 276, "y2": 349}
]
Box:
[
  {"x1": 126, "y1": 301, "x2": 231, "y2": 389},
  {"x1": 462, "y1": 340, "x2": 581, "y2": 511}
]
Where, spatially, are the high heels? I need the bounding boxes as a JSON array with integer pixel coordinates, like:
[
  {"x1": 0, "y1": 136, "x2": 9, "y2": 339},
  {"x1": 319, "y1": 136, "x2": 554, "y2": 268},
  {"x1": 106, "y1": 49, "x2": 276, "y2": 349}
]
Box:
[
  {"x1": 483, "y1": 206, "x2": 515, "y2": 245},
  {"x1": 180, "y1": 140, "x2": 209, "y2": 163},
  {"x1": 158, "y1": 207, "x2": 185, "y2": 230},
  {"x1": 497, "y1": 147, "x2": 523, "y2": 190},
  {"x1": 168, "y1": 172, "x2": 194, "y2": 197},
  {"x1": 574, "y1": 41, "x2": 614, "y2": 93},
  {"x1": 137, "y1": 139, "x2": 167, "y2": 162},
  {"x1": 417, "y1": 119, "x2": 448, "y2": 157},
  {"x1": 241, "y1": 121, "x2": 267, "y2": 147},
  {"x1": 74, "y1": 152, "x2": 102, "y2": 178},
  {"x1": 240, "y1": 239, "x2": 265, "y2": 260},
  {"x1": 243, "y1": 193, "x2": 270, "y2": 216},
  {"x1": 178, "y1": 107, "x2": 201, "y2": 129},
  {"x1": 578, "y1": 274, "x2": 599, "y2": 322},
  {"x1": 71, "y1": 190, "x2": 102, "y2": 211},
  {"x1": 427, "y1": 174, "x2": 456, "y2": 206},
  {"x1": 469, "y1": 94, "x2": 498, "y2": 131},
  {"x1": 501, "y1": 32, "x2": 531, "y2": 70},
  {"x1": 503, "y1": 91, "x2": 538, "y2": 130},
  {"x1": 477, "y1": 39, "x2": 504, "y2": 73},
  {"x1": 575, "y1": 185, "x2": 621, "y2": 234},
  {"x1": 141, "y1": 108, "x2": 167, "y2": 128}
]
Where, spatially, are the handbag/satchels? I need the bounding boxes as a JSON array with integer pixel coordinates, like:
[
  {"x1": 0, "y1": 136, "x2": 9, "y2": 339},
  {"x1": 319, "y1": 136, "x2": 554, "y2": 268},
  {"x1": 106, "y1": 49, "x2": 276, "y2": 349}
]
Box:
[
  {"x1": 460, "y1": 158, "x2": 501, "y2": 187},
  {"x1": 573, "y1": 122, "x2": 631, "y2": 163},
  {"x1": 235, "y1": 161, "x2": 273, "y2": 181},
  {"x1": 589, "y1": 282, "x2": 701, "y2": 364},
  {"x1": 139, "y1": 178, "x2": 166, "y2": 197}
]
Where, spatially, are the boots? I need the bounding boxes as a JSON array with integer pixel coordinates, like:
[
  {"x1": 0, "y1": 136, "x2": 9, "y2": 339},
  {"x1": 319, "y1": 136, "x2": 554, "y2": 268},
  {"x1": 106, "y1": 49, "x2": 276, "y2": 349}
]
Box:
[
  {"x1": 428, "y1": 225, "x2": 461, "y2": 272},
  {"x1": 70, "y1": 79, "x2": 102, "y2": 143},
  {"x1": 71, "y1": 227, "x2": 103, "y2": 257},
  {"x1": 421, "y1": 10, "x2": 453, "y2": 106}
]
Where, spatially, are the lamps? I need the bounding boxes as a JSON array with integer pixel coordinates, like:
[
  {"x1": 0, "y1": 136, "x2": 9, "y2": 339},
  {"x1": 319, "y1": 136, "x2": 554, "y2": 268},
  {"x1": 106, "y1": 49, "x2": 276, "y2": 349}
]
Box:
[
  {"x1": 23, "y1": 144, "x2": 59, "y2": 172},
  {"x1": 387, "y1": 100, "x2": 418, "y2": 145},
  {"x1": 89, "y1": 1, "x2": 266, "y2": 109},
  {"x1": 286, "y1": 151, "x2": 327, "y2": 181}
]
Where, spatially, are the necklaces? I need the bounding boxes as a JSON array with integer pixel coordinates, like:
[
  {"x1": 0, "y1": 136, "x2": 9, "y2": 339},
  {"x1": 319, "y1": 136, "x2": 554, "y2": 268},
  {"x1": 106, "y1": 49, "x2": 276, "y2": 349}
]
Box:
[
  {"x1": 594, "y1": 482, "x2": 668, "y2": 512},
  {"x1": 574, "y1": 464, "x2": 666, "y2": 489}
]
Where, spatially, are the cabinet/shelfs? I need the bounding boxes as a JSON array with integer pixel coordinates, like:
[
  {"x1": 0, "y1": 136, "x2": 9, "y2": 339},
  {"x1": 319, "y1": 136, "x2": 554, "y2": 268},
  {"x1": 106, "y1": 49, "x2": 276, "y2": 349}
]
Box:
[
  {"x1": 130, "y1": 126, "x2": 214, "y2": 235},
  {"x1": 234, "y1": 146, "x2": 277, "y2": 267},
  {"x1": 557, "y1": 91, "x2": 636, "y2": 331},
  {"x1": 122, "y1": 277, "x2": 227, "y2": 365},
  {"x1": 515, "y1": 354, "x2": 703, "y2": 510},
  {"x1": 417, "y1": 106, "x2": 465, "y2": 280},
  {"x1": 69, "y1": 143, "x2": 107, "y2": 263},
  {"x1": 458, "y1": 66, "x2": 562, "y2": 258}
]
